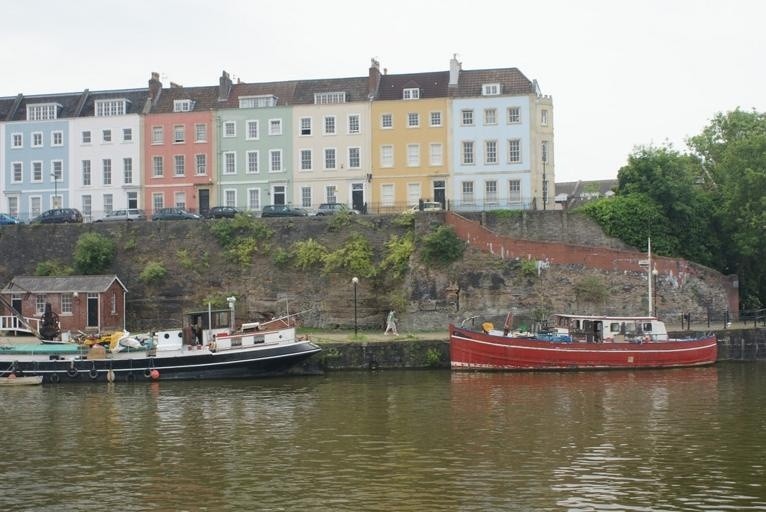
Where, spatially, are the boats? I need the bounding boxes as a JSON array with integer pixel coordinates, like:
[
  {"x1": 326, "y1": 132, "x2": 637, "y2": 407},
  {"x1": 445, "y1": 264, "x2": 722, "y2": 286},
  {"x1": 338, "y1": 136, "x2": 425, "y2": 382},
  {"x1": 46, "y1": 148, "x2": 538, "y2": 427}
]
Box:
[
  {"x1": 449, "y1": 236, "x2": 721, "y2": 370},
  {"x1": 0, "y1": 294, "x2": 323, "y2": 384},
  {"x1": 1, "y1": 375, "x2": 44, "y2": 386}
]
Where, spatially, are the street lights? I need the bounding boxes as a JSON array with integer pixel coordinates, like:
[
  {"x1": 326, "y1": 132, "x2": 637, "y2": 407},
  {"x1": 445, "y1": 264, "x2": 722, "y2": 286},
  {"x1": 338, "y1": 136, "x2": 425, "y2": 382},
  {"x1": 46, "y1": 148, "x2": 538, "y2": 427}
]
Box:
[
  {"x1": 352, "y1": 276, "x2": 360, "y2": 338},
  {"x1": 50, "y1": 173, "x2": 58, "y2": 209},
  {"x1": 652, "y1": 268, "x2": 659, "y2": 317}
]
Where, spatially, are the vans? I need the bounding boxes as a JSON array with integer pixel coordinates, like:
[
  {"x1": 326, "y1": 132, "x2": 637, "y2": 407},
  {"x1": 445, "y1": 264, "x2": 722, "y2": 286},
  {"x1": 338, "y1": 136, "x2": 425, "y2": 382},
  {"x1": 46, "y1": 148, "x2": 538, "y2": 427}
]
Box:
[
  {"x1": 261, "y1": 205, "x2": 309, "y2": 218},
  {"x1": 29, "y1": 208, "x2": 83, "y2": 224}
]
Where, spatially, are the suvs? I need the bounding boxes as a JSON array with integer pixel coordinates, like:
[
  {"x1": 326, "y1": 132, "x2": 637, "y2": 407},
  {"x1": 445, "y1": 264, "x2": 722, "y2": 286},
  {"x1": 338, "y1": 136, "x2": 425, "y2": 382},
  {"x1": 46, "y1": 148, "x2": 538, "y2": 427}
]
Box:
[
  {"x1": 208, "y1": 205, "x2": 252, "y2": 219},
  {"x1": 315, "y1": 201, "x2": 362, "y2": 217},
  {"x1": 403, "y1": 200, "x2": 444, "y2": 214},
  {"x1": 94, "y1": 208, "x2": 146, "y2": 223}
]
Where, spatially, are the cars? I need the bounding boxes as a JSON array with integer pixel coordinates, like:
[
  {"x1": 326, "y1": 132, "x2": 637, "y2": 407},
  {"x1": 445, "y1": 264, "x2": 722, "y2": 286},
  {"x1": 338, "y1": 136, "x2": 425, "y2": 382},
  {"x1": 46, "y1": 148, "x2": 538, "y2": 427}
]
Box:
[
  {"x1": 0, "y1": 214, "x2": 25, "y2": 224},
  {"x1": 152, "y1": 207, "x2": 201, "y2": 220}
]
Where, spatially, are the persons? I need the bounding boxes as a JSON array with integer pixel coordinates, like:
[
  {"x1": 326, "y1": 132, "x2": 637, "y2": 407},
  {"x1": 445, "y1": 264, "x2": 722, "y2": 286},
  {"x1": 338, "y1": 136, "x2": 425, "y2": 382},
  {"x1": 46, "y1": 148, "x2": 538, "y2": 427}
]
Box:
[{"x1": 382, "y1": 309, "x2": 400, "y2": 337}]
